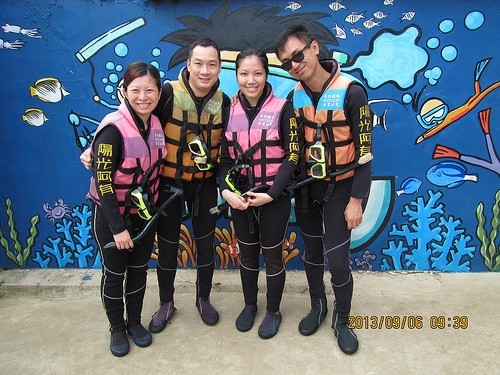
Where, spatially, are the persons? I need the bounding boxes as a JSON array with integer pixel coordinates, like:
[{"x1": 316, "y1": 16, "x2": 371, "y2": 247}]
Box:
[
  {"x1": 230, "y1": 25, "x2": 372, "y2": 354},
  {"x1": 218, "y1": 47, "x2": 300, "y2": 340},
  {"x1": 84, "y1": 62, "x2": 167, "y2": 357},
  {"x1": 80, "y1": 38, "x2": 231, "y2": 333}
]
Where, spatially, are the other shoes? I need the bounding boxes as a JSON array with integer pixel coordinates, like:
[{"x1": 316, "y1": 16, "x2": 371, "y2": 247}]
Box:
[
  {"x1": 330, "y1": 318, "x2": 358, "y2": 353},
  {"x1": 258, "y1": 310, "x2": 282, "y2": 338},
  {"x1": 236, "y1": 305, "x2": 257, "y2": 331},
  {"x1": 109, "y1": 330, "x2": 130, "y2": 356},
  {"x1": 148, "y1": 301, "x2": 175, "y2": 333},
  {"x1": 126, "y1": 323, "x2": 153, "y2": 347},
  {"x1": 195, "y1": 296, "x2": 219, "y2": 325},
  {"x1": 298, "y1": 308, "x2": 328, "y2": 335}
]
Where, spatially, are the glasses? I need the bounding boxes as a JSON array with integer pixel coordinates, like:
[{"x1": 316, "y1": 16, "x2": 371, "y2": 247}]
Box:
[
  {"x1": 280, "y1": 42, "x2": 310, "y2": 71},
  {"x1": 308, "y1": 141, "x2": 327, "y2": 179},
  {"x1": 225, "y1": 173, "x2": 241, "y2": 195},
  {"x1": 188, "y1": 139, "x2": 212, "y2": 170},
  {"x1": 130, "y1": 189, "x2": 152, "y2": 221}
]
